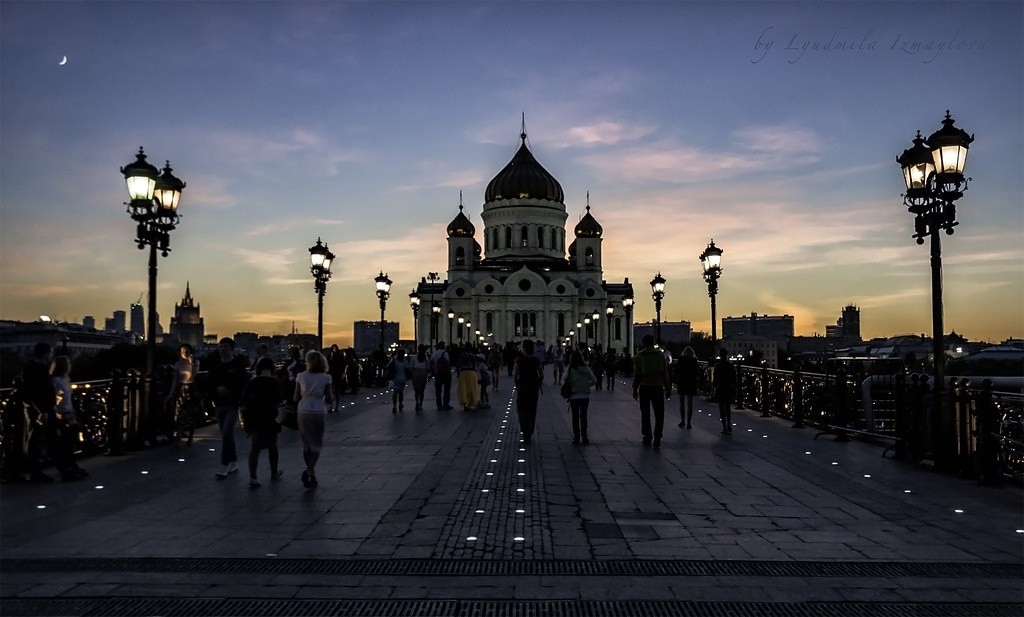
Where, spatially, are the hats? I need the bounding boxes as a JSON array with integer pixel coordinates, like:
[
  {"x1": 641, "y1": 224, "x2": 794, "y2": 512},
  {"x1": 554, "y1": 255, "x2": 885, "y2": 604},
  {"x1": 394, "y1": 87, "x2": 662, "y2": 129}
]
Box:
[
  {"x1": 476, "y1": 353, "x2": 485, "y2": 361},
  {"x1": 220, "y1": 338, "x2": 235, "y2": 350}
]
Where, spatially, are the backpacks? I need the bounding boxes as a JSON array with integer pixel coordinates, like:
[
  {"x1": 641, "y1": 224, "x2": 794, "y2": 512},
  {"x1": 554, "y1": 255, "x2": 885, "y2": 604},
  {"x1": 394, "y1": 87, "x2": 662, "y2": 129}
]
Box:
[
  {"x1": 412, "y1": 359, "x2": 428, "y2": 384},
  {"x1": 437, "y1": 352, "x2": 449, "y2": 378}
]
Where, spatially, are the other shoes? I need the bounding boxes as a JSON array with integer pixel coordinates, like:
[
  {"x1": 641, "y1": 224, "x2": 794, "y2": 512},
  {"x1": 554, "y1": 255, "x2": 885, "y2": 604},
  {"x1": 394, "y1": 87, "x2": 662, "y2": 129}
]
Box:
[
  {"x1": 228, "y1": 467, "x2": 239, "y2": 474},
  {"x1": 687, "y1": 423, "x2": 692, "y2": 429},
  {"x1": 679, "y1": 422, "x2": 685, "y2": 426},
  {"x1": 393, "y1": 408, "x2": 396, "y2": 413},
  {"x1": 643, "y1": 434, "x2": 653, "y2": 448},
  {"x1": 399, "y1": 405, "x2": 403, "y2": 411},
  {"x1": 271, "y1": 470, "x2": 283, "y2": 480},
  {"x1": 215, "y1": 467, "x2": 229, "y2": 479},
  {"x1": 653, "y1": 434, "x2": 663, "y2": 448},
  {"x1": 249, "y1": 478, "x2": 263, "y2": 487},
  {"x1": 415, "y1": 402, "x2": 422, "y2": 410},
  {"x1": 60, "y1": 464, "x2": 85, "y2": 481},
  {"x1": 301, "y1": 470, "x2": 317, "y2": 488},
  {"x1": 720, "y1": 426, "x2": 733, "y2": 433},
  {"x1": 443, "y1": 405, "x2": 453, "y2": 409},
  {"x1": 438, "y1": 406, "x2": 443, "y2": 409},
  {"x1": 345, "y1": 389, "x2": 352, "y2": 393}
]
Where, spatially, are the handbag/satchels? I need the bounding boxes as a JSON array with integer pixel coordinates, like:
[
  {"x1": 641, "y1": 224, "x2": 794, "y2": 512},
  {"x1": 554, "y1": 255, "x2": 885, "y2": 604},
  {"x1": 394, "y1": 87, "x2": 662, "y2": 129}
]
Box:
[
  {"x1": 275, "y1": 392, "x2": 299, "y2": 431},
  {"x1": 583, "y1": 366, "x2": 591, "y2": 394},
  {"x1": 386, "y1": 358, "x2": 397, "y2": 380},
  {"x1": 237, "y1": 403, "x2": 249, "y2": 432},
  {"x1": 561, "y1": 373, "x2": 568, "y2": 399}
]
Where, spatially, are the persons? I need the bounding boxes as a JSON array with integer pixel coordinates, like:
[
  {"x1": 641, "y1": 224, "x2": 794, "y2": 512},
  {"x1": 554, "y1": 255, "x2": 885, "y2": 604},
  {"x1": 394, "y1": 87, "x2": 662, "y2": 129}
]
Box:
[
  {"x1": 22, "y1": 339, "x2": 675, "y2": 488},
  {"x1": 673, "y1": 347, "x2": 703, "y2": 429},
  {"x1": 713, "y1": 348, "x2": 737, "y2": 435},
  {"x1": 634, "y1": 335, "x2": 670, "y2": 448}
]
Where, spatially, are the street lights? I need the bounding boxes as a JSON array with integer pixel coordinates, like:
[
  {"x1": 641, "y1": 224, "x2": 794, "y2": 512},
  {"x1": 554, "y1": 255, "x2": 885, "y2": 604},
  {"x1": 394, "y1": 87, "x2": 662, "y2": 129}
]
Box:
[
  {"x1": 621, "y1": 292, "x2": 634, "y2": 351},
  {"x1": 576, "y1": 319, "x2": 582, "y2": 344},
  {"x1": 475, "y1": 328, "x2": 480, "y2": 347},
  {"x1": 121, "y1": 147, "x2": 187, "y2": 445},
  {"x1": 374, "y1": 272, "x2": 393, "y2": 345},
  {"x1": 432, "y1": 300, "x2": 442, "y2": 345},
  {"x1": 569, "y1": 329, "x2": 574, "y2": 347},
  {"x1": 699, "y1": 237, "x2": 723, "y2": 366},
  {"x1": 583, "y1": 314, "x2": 591, "y2": 345},
  {"x1": 309, "y1": 238, "x2": 334, "y2": 353},
  {"x1": 650, "y1": 272, "x2": 667, "y2": 347},
  {"x1": 565, "y1": 335, "x2": 570, "y2": 346},
  {"x1": 895, "y1": 110, "x2": 974, "y2": 464},
  {"x1": 479, "y1": 335, "x2": 484, "y2": 346},
  {"x1": 466, "y1": 319, "x2": 472, "y2": 342},
  {"x1": 592, "y1": 309, "x2": 600, "y2": 346},
  {"x1": 458, "y1": 313, "x2": 464, "y2": 343},
  {"x1": 447, "y1": 308, "x2": 455, "y2": 343},
  {"x1": 409, "y1": 289, "x2": 421, "y2": 354},
  {"x1": 606, "y1": 302, "x2": 615, "y2": 348}
]
